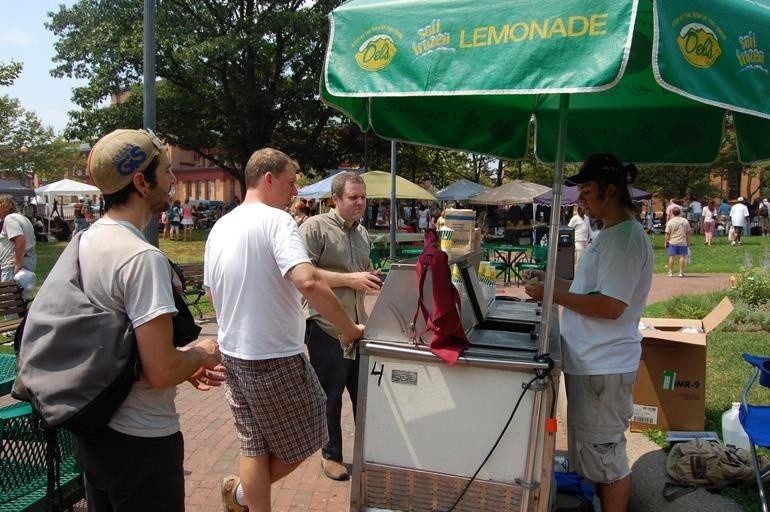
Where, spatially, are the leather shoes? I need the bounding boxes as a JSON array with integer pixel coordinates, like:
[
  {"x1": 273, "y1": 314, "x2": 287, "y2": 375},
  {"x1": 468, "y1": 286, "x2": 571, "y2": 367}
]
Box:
[
  {"x1": 320, "y1": 454, "x2": 350, "y2": 481},
  {"x1": 221, "y1": 474, "x2": 249, "y2": 512}
]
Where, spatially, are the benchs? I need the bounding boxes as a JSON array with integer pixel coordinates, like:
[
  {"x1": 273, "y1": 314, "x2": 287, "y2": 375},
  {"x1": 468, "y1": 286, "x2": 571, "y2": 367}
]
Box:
[
  {"x1": 641, "y1": 218, "x2": 665, "y2": 232},
  {"x1": 171, "y1": 262, "x2": 205, "y2": 318},
  {"x1": 481, "y1": 248, "x2": 506, "y2": 286},
  {"x1": 369, "y1": 248, "x2": 423, "y2": 272},
  {"x1": 515, "y1": 246, "x2": 549, "y2": 288},
  {"x1": 0, "y1": 279, "x2": 35, "y2": 333},
  {"x1": 0, "y1": 401, "x2": 86, "y2": 512}
]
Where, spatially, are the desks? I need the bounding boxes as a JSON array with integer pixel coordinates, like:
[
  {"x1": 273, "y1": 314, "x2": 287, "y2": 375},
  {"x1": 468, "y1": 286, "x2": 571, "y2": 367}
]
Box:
[
  {"x1": 401, "y1": 249, "x2": 423, "y2": 255},
  {"x1": 507, "y1": 223, "x2": 547, "y2": 247},
  {"x1": 492, "y1": 246, "x2": 527, "y2": 282},
  {"x1": 0, "y1": 353, "x2": 17, "y2": 397}
]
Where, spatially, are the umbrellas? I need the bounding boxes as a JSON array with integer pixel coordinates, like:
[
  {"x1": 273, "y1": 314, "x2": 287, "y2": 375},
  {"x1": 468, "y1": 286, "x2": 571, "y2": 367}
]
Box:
[
  {"x1": 297, "y1": 169, "x2": 438, "y2": 201},
  {"x1": 320, "y1": 0, "x2": 770, "y2": 512}
]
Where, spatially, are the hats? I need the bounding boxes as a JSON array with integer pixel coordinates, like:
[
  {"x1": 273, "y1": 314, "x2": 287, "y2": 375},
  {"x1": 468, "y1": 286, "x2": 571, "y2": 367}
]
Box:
[
  {"x1": 563, "y1": 153, "x2": 624, "y2": 187},
  {"x1": 737, "y1": 197, "x2": 744, "y2": 202},
  {"x1": 87, "y1": 126, "x2": 160, "y2": 195}
]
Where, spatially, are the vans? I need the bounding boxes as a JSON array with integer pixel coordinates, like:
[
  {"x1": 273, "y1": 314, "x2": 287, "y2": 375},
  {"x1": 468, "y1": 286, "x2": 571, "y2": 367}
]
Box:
[{"x1": 11, "y1": 194, "x2": 30, "y2": 205}]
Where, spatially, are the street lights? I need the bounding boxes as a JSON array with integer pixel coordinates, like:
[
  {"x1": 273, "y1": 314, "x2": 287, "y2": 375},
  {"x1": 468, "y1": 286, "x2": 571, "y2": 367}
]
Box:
[{"x1": 20, "y1": 145, "x2": 28, "y2": 213}]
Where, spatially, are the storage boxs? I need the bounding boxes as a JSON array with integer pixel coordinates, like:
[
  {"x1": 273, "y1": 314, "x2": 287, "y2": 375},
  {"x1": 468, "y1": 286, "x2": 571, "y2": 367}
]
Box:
[
  {"x1": 630, "y1": 297, "x2": 734, "y2": 433},
  {"x1": 440, "y1": 209, "x2": 482, "y2": 254}
]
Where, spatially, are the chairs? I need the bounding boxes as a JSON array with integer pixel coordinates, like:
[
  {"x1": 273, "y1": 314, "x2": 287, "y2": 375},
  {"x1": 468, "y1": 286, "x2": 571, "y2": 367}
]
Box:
[
  {"x1": 158, "y1": 213, "x2": 218, "y2": 237},
  {"x1": 738, "y1": 353, "x2": 770, "y2": 511}
]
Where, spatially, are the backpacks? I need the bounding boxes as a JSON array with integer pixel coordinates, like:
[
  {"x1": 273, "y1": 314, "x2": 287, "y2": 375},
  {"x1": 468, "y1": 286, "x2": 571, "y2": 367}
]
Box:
[
  {"x1": 758, "y1": 202, "x2": 768, "y2": 217},
  {"x1": 662, "y1": 439, "x2": 770, "y2": 503}
]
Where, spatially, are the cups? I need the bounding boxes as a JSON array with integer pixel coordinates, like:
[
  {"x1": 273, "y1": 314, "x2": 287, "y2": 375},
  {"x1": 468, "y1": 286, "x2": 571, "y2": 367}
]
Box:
[
  {"x1": 450, "y1": 261, "x2": 499, "y2": 329},
  {"x1": 435, "y1": 230, "x2": 454, "y2": 251}
]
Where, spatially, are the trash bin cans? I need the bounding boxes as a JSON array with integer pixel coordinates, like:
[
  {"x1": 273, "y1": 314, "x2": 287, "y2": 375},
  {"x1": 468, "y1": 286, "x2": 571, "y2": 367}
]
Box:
[
  {"x1": 654, "y1": 220, "x2": 661, "y2": 230},
  {"x1": 557, "y1": 226, "x2": 576, "y2": 282}
]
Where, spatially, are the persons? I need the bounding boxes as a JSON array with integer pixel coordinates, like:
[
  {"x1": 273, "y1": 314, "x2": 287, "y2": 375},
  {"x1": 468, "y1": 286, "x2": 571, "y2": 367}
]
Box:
[
  {"x1": 31, "y1": 195, "x2": 104, "y2": 242},
  {"x1": 202, "y1": 148, "x2": 365, "y2": 511},
  {"x1": 162, "y1": 196, "x2": 240, "y2": 242},
  {"x1": 285, "y1": 198, "x2": 331, "y2": 227},
  {"x1": 75, "y1": 128, "x2": 228, "y2": 512},
  {"x1": 523, "y1": 153, "x2": 654, "y2": 510},
  {"x1": 0, "y1": 194, "x2": 36, "y2": 345},
  {"x1": 567, "y1": 207, "x2": 592, "y2": 261},
  {"x1": 298, "y1": 170, "x2": 381, "y2": 479},
  {"x1": 664, "y1": 207, "x2": 691, "y2": 278},
  {"x1": 366, "y1": 200, "x2": 437, "y2": 246},
  {"x1": 664, "y1": 197, "x2": 770, "y2": 245}
]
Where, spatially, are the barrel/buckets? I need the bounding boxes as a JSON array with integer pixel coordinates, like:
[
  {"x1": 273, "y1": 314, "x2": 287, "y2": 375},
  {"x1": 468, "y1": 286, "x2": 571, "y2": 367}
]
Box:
[
  {"x1": 442, "y1": 208, "x2": 477, "y2": 249},
  {"x1": 452, "y1": 264, "x2": 461, "y2": 279},
  {"x1": 720, "y1": 402, "x2": 760, "y2": 452},
  {"x1": 483, "y1": 266, "x2": 496, "y2": 281},
  {"x1": 478, "y1": 260, "x2": 490, "y2": 277},
  {"x1": 727, "y1": 229, "x2": 739, "y2": 242}
]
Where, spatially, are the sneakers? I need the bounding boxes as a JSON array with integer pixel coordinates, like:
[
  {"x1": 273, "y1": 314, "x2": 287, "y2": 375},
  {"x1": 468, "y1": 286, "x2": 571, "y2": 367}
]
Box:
[
  {"x1": 2, "y1": 330, "x2": 16, "y2": 339},
  {"x1": 667, "y1": 271, "x2": 684, "y2": 277},
  {"x1": 731, "y1": 241, "x2": 743, "y2": 246},
  {"x1": 704, "y1": 240, "x2": 711, "y2": 246}
]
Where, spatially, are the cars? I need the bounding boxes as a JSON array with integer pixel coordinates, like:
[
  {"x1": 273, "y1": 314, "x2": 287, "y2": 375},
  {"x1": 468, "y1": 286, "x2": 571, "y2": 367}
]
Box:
[
  {"x1": 639, "y1": 216, "x2": 766, "y2": 236},
  {"x1": 91, "y1": 200, "x2": 106, "y2": 211},
  {"x1": 192, "y1": 200, "x2": 224, "y2": 218}
]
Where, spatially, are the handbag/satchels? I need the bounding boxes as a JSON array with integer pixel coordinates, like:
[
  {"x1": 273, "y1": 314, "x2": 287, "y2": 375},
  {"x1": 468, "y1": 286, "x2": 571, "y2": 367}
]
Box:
[{"x1": 10, "y1": 228, "x2": 201, "y2": 437}]
